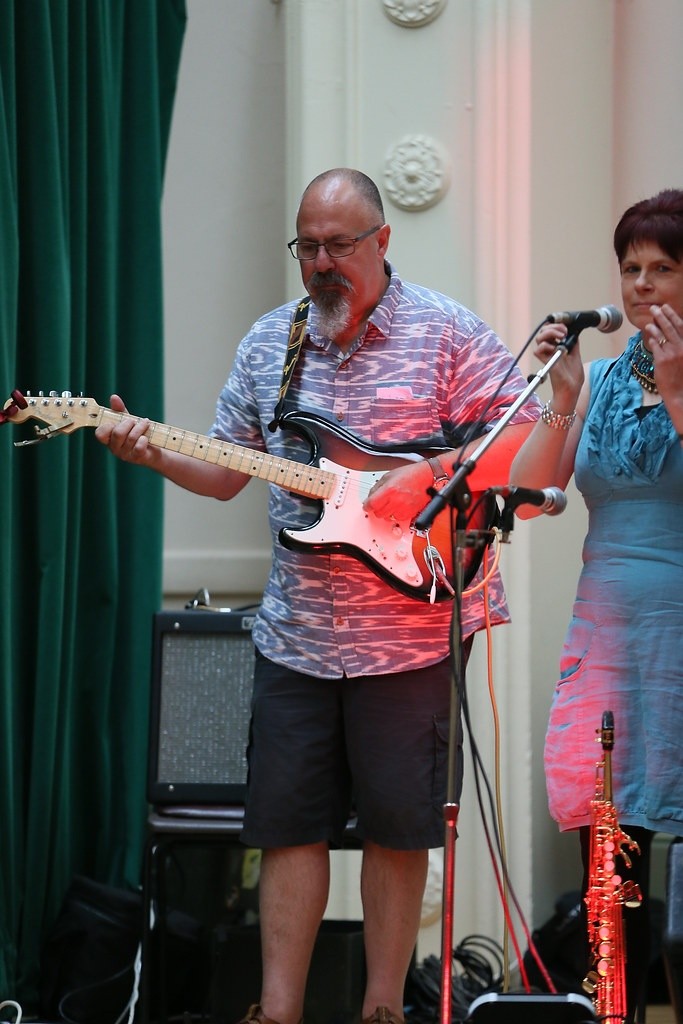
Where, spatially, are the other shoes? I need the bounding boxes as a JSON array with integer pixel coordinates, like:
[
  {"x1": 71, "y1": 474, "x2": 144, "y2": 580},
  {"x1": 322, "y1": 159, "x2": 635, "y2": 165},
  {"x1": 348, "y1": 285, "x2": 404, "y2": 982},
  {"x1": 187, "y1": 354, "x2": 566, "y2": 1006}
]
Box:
[
  {"x1": 237, "y1": 1004, "x2": 304, "y2": 1024},
  {"x1": 363, "y1": 1007, "x2": 406, "y2": 1024}
]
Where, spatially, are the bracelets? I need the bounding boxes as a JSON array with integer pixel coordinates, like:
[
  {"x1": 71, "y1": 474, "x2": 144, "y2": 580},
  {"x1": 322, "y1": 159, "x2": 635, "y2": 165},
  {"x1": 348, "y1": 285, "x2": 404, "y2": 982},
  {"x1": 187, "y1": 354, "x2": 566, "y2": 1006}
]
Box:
[{"x1": 541, "y1": 399, "x2": 577, "y2": 430}]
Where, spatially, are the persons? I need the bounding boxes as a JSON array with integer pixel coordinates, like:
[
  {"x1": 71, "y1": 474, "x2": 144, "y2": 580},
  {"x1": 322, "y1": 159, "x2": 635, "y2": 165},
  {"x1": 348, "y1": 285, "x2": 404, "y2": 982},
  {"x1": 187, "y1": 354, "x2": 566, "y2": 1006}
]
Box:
[
  {"x1": 508, "y1": 188, "x2": 683, "y2": 1024},
  {"x1": 95, "y1": 167, "x2": 544, "y2": 1024}
]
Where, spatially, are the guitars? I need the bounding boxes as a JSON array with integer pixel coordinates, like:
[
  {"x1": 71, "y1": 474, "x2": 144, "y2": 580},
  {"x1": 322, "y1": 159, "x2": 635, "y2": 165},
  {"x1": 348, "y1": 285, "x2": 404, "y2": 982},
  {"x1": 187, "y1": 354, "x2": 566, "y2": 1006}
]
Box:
[{"x1": 0, "y1": 389, "x2": 496, "y2": 603}]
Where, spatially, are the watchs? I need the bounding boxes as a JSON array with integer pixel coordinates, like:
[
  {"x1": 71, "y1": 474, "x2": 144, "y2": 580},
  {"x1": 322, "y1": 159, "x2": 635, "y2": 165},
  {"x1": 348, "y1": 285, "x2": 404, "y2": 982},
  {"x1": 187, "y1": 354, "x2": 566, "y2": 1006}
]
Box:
[{"x1": 425, "y1": 458, "x2": 451, "y2": 493}]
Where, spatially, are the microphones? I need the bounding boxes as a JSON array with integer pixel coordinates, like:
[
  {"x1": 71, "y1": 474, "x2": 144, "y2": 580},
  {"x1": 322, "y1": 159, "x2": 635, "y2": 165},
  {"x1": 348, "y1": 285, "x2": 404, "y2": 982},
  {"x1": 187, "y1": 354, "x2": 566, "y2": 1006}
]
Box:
[
  {"x1": 489, "y1": 484, "x2": 568, "y2": 516},
  {"x1": 546, "y1": 303, "x2": 623, "y2": 333}
]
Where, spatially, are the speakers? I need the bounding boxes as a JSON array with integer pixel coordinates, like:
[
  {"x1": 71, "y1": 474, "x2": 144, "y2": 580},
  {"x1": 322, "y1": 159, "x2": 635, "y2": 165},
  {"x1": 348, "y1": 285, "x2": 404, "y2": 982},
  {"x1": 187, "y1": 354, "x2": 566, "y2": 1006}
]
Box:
[{"x1": 153, "y1": 610, "x2": 257, "y2": 805}]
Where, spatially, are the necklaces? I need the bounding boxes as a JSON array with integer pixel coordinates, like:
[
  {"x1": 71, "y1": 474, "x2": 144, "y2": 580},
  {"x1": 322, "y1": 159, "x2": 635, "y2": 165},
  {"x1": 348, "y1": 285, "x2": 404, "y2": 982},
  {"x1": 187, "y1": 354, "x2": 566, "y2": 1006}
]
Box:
[{"x1": 632, "y1": 340, "x2": 658, "y2": 396}]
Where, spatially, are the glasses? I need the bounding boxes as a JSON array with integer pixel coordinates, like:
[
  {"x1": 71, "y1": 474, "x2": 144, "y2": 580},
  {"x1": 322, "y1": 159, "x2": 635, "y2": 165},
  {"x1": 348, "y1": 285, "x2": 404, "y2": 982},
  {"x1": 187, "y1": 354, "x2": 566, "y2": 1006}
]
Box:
[{"x1": 288, "y1": 225, "x2": 380, "y2": 260}]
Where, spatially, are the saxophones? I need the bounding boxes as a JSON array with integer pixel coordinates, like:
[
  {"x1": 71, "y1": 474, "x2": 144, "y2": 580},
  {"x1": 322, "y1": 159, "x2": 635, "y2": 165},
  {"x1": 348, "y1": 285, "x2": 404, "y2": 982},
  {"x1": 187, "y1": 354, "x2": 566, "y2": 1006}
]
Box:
[{"x1": 583, "y1": 710, "x2": 642, "y2": 1024}]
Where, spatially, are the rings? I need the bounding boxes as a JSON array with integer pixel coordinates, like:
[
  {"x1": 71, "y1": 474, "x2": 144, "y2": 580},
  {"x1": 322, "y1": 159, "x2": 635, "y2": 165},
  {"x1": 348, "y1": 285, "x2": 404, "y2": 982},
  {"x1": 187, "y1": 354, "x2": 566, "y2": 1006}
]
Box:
[
  {"x1": 658, "y1": 336, "x2": 669, "y2": 347},
  {"x1": 391, "y1": 514, "x2": 396, "y2": 521}
]
